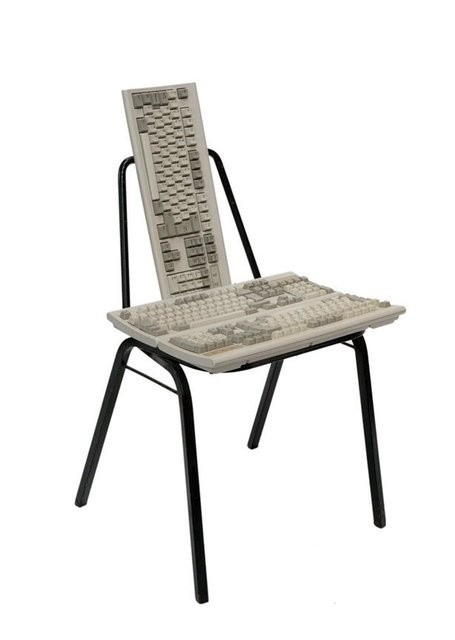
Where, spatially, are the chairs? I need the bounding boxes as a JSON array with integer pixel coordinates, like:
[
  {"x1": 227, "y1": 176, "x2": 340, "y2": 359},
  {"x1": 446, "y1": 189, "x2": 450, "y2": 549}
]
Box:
[{"x1": 74, "y1": 148, "x2": 387, "y2": 604}]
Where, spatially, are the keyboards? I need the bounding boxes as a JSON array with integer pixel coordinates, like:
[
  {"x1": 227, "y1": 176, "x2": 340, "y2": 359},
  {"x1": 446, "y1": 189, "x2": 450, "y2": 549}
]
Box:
[
  {"x1": 159, "y1": 291, "x2": 407, "y2": 375},
  {"x1": 121, "y1": 81, "x2": 232, "y2": 299},
  {"x1": 108, "y1": 271, "x2": 343, "y2": 348}
]
